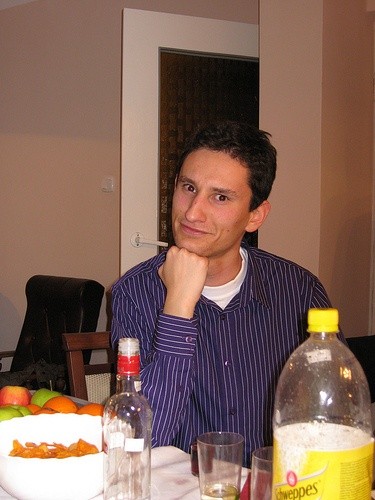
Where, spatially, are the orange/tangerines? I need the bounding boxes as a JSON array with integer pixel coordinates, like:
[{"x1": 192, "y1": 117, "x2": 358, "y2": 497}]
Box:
[{"x1": 23, "y1": 396, "x2": 115, "y2": 417}]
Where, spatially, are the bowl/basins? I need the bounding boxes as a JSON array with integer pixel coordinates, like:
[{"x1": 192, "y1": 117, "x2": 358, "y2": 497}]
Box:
[{"x1": 0, "y1": 413, "x2": 102, "y2": 499}]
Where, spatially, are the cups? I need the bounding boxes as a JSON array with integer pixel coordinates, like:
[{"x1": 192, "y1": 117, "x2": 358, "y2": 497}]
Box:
[
  {"x1": 197, "y1": 432, "x2": 244, "y2": 500},
  {"x1": 191, "y1": 443, "x2": 199, "y2": 477},
  {"x1": 249, "y1": 446, "x2": 272, "y2": 500}
]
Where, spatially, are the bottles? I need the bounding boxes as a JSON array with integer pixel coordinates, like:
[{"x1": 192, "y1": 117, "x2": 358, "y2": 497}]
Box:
[
  {"x1": 102, "y1": 338, "x2": 152, "y2": 500},
  {"x1": 272, "y1": 308, "x2": 374, "y2": 500}
]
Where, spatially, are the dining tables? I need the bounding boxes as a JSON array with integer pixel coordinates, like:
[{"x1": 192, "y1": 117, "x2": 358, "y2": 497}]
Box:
[{"x1": 1, "y1": 389, "x2": 274, "y2": 500}]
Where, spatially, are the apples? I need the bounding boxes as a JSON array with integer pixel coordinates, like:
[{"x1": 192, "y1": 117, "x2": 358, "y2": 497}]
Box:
[{"x1": 0, "y1": 380, "x2": 63, "y2": 422}]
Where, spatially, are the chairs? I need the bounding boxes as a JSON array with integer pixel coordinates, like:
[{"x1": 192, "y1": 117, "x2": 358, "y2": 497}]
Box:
[
  {"x1": 0, "y1": 274, "x2": 106, "y2": 395},
  {"x1": 343, "y1": 333, "x2": 374, "y2": 396},
  {"x1": 63, "y1": 330, "x2": 119, "y2": 413}
]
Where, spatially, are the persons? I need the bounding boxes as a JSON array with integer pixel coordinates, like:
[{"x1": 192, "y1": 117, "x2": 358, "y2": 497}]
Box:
[{"x1": 106, "y1": 122, "x2": 349, "y2": 469}]
